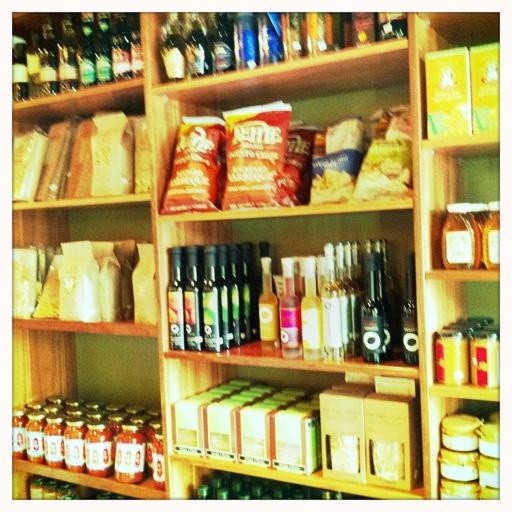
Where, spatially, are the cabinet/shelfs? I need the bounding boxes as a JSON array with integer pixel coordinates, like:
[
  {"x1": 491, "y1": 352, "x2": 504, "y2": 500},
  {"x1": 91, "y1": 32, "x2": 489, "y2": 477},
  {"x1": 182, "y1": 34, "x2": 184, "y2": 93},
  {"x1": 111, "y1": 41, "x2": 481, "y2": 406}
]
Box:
[
  {"x1": 13, "y1": 12, "x2": 169, "y2": 499},
  {"x1": 408, "y1": 12, "x2": 500, "y2": 500},
  {"x1": 140, "y1": 13, "x2": 432, "y2": 500}
]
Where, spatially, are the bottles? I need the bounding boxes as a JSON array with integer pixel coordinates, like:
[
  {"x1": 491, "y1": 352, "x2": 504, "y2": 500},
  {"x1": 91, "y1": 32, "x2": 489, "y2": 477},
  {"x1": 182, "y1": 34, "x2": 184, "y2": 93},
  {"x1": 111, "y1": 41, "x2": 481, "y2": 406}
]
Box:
[
  {"x1": 195, "y1": 472, "x2": 344, "y2": 500},
  {"x1": 12, "y1": 11, "x2": 143, "y2": 102},
  {"x1": 434, "y1": 317, "x2": 499, "y2": 387},
  {"x1": 30, "y1": 477, "x2": 127, "y2": 499},
  {"x1": 12, "y1": 395, "x2": 167, "y2": 489},
  {"x1": 441, "y1": 201, "x2": 500, "y2": 270},
  {"x1": 158, "y1": 12, "x2": 407, "y2": 82},
  {"x1": 167, "y1": 241, "x2": 418, "y2": 365}
]
